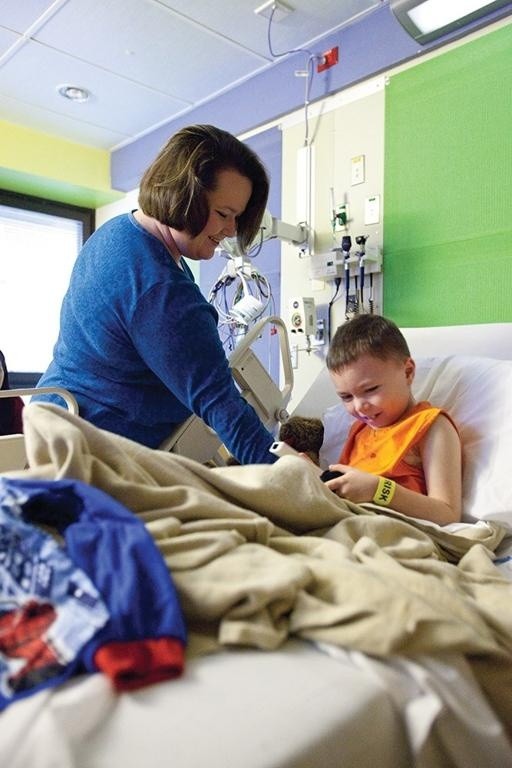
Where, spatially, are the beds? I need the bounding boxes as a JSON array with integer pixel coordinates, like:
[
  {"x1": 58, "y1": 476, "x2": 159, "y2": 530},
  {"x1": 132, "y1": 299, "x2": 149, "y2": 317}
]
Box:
[{"x1": 3, "y1": 319, "x2": 509, "y2": 766}]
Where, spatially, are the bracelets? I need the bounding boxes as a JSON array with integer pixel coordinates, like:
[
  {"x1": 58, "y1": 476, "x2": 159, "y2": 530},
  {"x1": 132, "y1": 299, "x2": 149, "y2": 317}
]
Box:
[{"x1": 374, "y1": 477, "x2": 396, "y2": 508}]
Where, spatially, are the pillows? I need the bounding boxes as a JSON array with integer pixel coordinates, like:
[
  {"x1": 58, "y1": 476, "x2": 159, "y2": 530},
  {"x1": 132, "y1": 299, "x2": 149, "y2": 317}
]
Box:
[{"x1": 313, "y1": 348, "x2": 509, "y2": 529}]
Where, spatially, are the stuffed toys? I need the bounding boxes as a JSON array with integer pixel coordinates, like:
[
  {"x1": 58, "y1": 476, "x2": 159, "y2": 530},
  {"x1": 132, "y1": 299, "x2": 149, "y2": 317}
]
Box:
[{"x1": 226, "y1": 416, "x2": 324, "y2": 466}]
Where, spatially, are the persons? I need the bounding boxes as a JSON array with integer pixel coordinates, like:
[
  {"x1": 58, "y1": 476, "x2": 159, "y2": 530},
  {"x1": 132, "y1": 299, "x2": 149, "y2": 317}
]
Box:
[
  {"x1": 323, "y1": 315, "x2": 463, "y2": 527},
  {"x1": 0, "y1": 349, "x2": 25, "y2": 436},
  {"x1": 20, "y1": 125, "x2": 279, "y2": 465}
]
